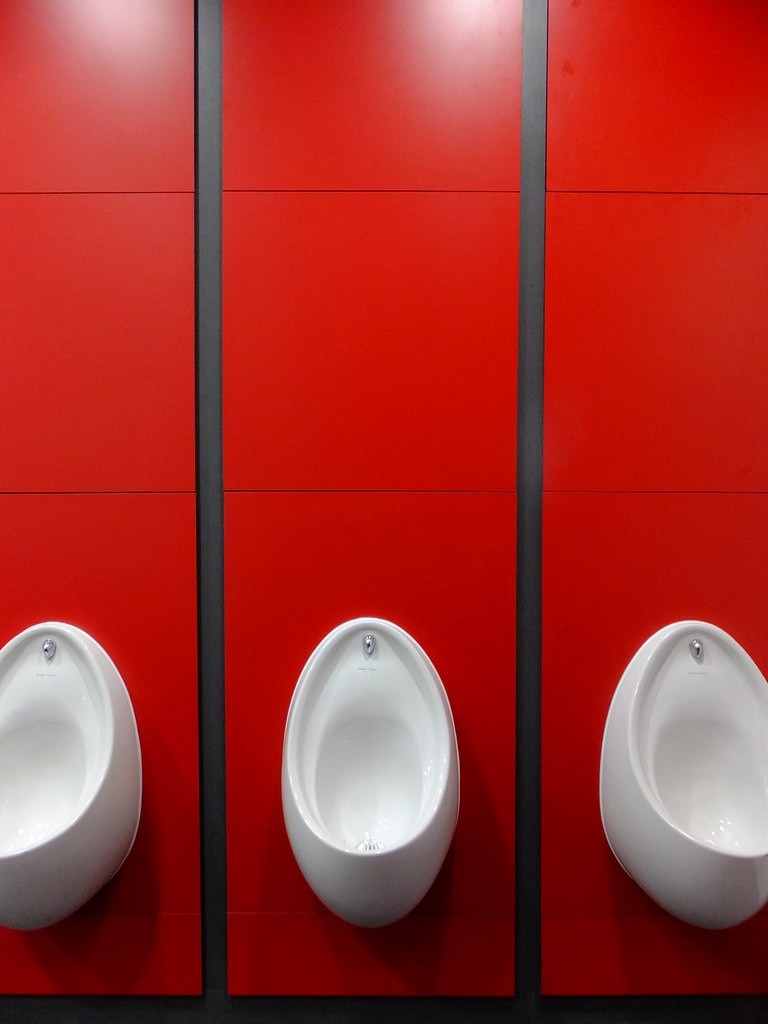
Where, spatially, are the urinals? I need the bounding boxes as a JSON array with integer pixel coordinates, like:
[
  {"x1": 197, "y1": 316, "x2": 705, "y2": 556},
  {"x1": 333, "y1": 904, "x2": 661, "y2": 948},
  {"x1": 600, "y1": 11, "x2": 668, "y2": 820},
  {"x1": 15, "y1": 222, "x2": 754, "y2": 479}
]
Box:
[
  {"x1": 0, "y1": 620, "x2": 143, "y2": 931},
  {"x1": 598, "y1": 618, "x2": 768, "y2": 928},
  {"x1": 280, "y1": 616, "x2": 462, "y2": 930}
]
